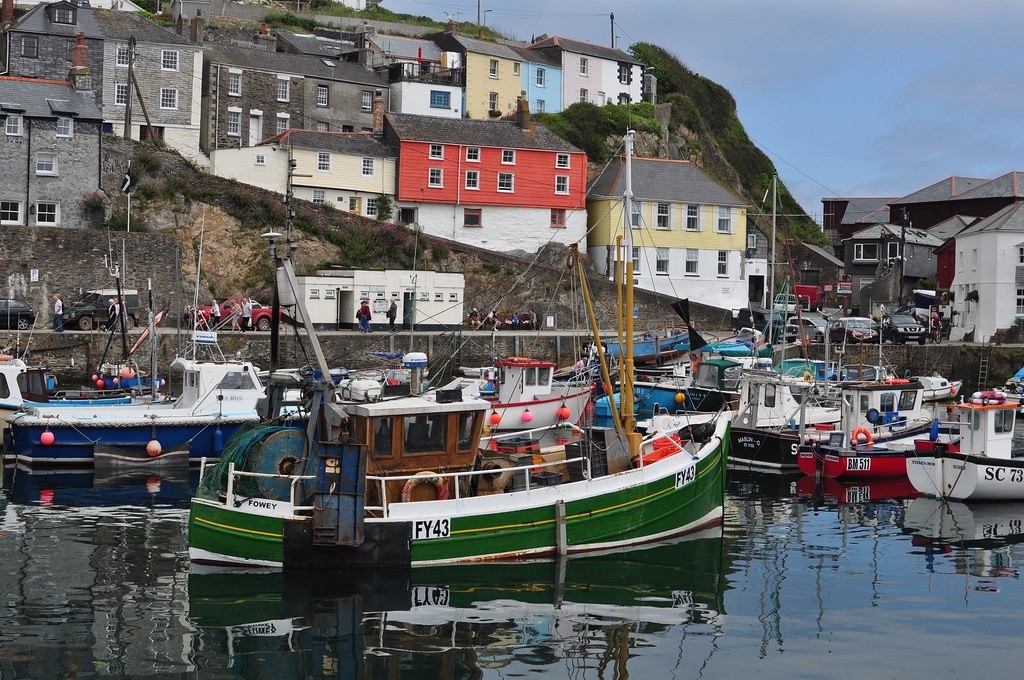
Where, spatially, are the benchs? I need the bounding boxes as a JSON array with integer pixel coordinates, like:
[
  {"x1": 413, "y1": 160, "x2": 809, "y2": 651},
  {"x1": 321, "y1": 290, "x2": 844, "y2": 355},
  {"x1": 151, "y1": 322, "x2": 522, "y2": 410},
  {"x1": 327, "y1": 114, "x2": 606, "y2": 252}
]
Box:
[{"x1": 480, "y1": 313, "x2": 540, "y2": 330}]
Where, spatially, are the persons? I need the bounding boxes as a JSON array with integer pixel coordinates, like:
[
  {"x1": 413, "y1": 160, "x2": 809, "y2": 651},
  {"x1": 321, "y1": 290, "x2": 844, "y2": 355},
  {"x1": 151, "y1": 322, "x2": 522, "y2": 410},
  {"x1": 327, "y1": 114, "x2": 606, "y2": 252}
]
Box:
[
  {"x1": 530, "y1": 308, "x2": 537, "y2": 329},
  {"x1": 242, "y1": 297, "x2": 251, "y2": 332},
  {"x1": 390, "y1": 299, "x2": 396, "y2": 332},
  {"x1": 489, "y1": 308, "x2": 501, "y2": 331},
  {"x1": 470, "y1": 308, "x2": 480, "y2": 331},
  {"x1": 932, "y1": 313, "x2": 942, "y2": 335},
  {"x1": 210, "y1": 300, "x2": 222, "y2": 331},
  {"x1": 358, "y1": 301, "x2": 372, "y2": 333},
  {"x1": 574, "y1": 357, "x2": 587, "y2": 381},
  {"x1": 513, "y1": 313, "x2": 519, "y2": 328},
  {"x1": 108, "y1": 296, "x2": 128, "y2": 333},
  {"x1": 231, "y1": 300, "x2": 242, "y2": 333},
  {"x1": 54, "y1": 296, "x2": 63, "y2": 332},
  {"x1": 690, "y1": 354, "x2": 702, "y2": 380}
]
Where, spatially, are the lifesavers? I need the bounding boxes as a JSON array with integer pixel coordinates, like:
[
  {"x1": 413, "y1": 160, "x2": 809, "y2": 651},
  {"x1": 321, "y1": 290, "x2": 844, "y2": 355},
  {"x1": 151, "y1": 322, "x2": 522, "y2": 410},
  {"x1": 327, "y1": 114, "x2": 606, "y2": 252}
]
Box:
[
  {"x1": 853, "y1": 427, "x2": 873, "y2": 448},
  {"x1": 507, "y1": 356, "x2": 532, "y2": 362},
  {"x1": 679, "y1": 424, "x2": 706, "y2": 439},
  {"x1": 969, "y1": 399, "x2": 1004, "y2": 405},
  {"x1": 401, "y1": 471, "x2": 448, "y2": 502},
  {"x1": 694, "y1": 423, "x2": 714, "y2": 442},
  {"x1": 804, "y1": 372, "x2": 812, "y2": 380},
  {"x1": 885, "y1": 379, "x2": 909, "y2": 384},
  {"x1": 972, "y1": 391, "x2": 1007, "y2": 400}
]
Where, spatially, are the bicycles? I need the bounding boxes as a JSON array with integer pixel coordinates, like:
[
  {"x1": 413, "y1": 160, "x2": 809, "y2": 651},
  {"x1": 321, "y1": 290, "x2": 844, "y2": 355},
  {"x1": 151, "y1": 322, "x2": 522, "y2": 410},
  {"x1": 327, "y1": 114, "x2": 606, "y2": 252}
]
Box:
[{"x1": 928, "y1": 326, "x2": 942, "y2": 344}]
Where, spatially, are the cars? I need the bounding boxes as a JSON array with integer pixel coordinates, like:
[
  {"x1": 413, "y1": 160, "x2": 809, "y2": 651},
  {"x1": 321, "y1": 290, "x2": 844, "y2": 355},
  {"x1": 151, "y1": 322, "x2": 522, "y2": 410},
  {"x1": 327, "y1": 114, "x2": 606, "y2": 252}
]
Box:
[
  {"x1": 731, "y1": 285, "x2": 928, "y2": 345},
  {"x1": 0, "y1": 299, "x2": 35, "y2": 330}
]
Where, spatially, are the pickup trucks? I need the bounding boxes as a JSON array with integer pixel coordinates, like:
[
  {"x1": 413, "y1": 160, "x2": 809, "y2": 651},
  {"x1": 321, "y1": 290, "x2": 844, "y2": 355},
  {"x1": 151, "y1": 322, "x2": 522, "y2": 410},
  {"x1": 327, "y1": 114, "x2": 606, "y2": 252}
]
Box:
[
  {"x1": 183, "y1": 296, "x2": 282, "y2": 330},
  {"x1": 62, "y1": 288, "x2": 141, "y2": 331}
]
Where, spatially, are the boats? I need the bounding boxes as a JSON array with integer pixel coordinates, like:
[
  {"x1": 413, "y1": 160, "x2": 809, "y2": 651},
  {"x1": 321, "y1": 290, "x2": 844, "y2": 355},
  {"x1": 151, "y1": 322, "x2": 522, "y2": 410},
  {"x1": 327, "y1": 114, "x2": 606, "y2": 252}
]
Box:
[
  {"x1": 1, "y1": 333, "x2": 1024, "y2": 498},
  {"x1": 785, "y1": 476, "x2": 914, "y2": 509}
]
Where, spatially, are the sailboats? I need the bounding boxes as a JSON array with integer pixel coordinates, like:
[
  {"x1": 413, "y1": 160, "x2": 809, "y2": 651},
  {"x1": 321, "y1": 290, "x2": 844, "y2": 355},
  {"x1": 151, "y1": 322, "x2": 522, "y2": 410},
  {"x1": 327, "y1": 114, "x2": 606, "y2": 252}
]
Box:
[{"x1": 186, "y1": 128, "x2": 738, "y2": 569}]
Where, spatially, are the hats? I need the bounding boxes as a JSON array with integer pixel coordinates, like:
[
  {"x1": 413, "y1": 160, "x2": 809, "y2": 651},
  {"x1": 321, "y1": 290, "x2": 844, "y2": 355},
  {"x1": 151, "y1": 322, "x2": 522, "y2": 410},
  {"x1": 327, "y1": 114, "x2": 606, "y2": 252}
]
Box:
[{"x1": 473, "y1": 308, "x2": 477, "y2": 311}]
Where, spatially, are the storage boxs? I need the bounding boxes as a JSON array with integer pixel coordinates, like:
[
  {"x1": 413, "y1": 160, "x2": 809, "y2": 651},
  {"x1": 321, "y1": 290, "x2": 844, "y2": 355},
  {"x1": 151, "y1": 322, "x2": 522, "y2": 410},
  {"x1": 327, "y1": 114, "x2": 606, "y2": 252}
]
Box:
[
  {"x1": 816, "y1": 424, "x2": 833, "y2": 430},
  {"x1": 915, "y1": 439, "x2": 936, "y2": 450},
  {"x1": 636, "y1": 447, "x2": 680, "y2": 468},
  {"x1": 496, "y1": 437, "x2": 607, "y2": 489}
]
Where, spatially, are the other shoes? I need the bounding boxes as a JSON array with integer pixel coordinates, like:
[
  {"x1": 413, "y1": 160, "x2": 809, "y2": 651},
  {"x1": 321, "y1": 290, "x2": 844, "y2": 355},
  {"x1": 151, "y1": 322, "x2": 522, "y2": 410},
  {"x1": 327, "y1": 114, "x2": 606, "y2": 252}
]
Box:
[
  {"x1": 253, "y1": 327, "x2": 256, "y2": 332},
  {"x1": 239, "y1": 331, "x2": 245, "y2": 335},
  {"x1": 494, "y1": 329, "x2": 498, "y2": 331}
]
